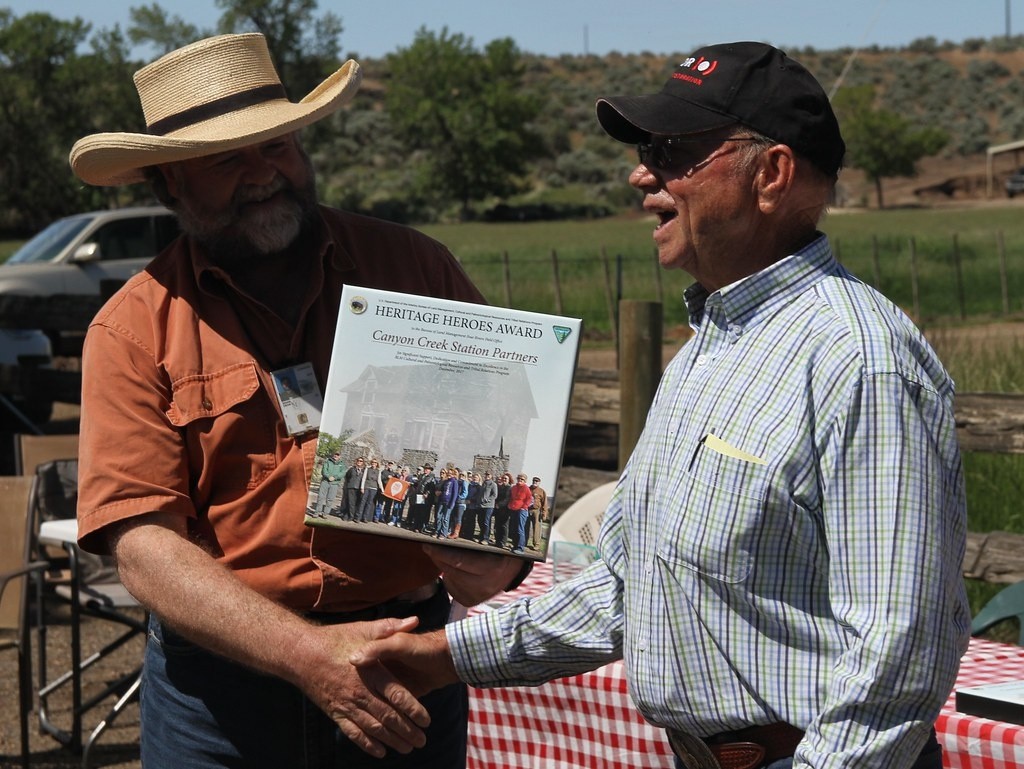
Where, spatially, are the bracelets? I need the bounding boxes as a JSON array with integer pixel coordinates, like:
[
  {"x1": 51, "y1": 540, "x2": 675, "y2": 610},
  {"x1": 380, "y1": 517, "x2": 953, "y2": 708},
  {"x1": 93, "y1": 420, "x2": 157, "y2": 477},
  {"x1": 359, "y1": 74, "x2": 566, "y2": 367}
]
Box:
[{"x1": 503, "y1": 559, "x2": 534, "y2": 592}]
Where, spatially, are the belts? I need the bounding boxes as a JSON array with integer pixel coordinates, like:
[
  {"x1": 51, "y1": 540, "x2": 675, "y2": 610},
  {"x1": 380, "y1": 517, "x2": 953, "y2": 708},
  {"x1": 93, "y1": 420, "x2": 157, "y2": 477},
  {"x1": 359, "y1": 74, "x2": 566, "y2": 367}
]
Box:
[
  {"x1": 297, "y1": 577, "x2": 442, "y2": 626},
  {"x1": 664, "y1": 721, "x2": 938, "y2": 768}
]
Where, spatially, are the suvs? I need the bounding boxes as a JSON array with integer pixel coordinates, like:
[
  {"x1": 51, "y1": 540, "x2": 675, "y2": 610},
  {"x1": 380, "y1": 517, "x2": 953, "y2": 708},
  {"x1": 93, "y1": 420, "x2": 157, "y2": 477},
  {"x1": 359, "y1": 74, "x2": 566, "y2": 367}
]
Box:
[
  {"x1": 0, "y1": 207, "x2": 182, "y2": 324},
  {"x1": 1006, "y1": 175, "x2": 1024, "y2": 197}
]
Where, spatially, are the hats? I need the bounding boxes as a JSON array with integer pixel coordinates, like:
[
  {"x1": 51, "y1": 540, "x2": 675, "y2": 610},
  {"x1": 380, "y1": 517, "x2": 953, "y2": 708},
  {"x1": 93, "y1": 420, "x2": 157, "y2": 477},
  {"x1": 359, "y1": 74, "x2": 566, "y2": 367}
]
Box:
[
  {"x1": 417, "y1": 466, "x2": 424, "y2": 472},
  {"x1": 421, "y1": 463, "x2": 434, "y2": 471},
  {"x1": 594, "y1": 41, "x2": 845, "y2": 177},
  {"x1": 68, "y1": 32, "x2": 362, "y2": 188}
]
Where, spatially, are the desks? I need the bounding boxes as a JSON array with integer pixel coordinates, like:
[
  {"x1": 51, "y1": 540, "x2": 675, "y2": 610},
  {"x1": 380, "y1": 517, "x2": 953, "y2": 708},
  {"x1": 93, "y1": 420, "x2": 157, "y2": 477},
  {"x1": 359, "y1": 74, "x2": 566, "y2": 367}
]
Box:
[{"x1": 445, "y1": 558, "x2": 1024, "y2": 768}]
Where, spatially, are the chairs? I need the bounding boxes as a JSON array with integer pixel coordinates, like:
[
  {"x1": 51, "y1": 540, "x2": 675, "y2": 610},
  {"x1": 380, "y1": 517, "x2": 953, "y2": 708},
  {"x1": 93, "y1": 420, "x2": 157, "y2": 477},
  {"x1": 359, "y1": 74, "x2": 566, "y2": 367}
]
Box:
[{"x1": 20, "y1": 458, "x2": 160, "y2": 768}]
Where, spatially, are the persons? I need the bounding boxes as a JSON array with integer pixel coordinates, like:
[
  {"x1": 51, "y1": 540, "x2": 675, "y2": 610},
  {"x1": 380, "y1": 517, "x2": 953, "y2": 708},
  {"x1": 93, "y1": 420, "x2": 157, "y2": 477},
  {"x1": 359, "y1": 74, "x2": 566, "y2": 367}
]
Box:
[
  {"x1": 347, "y1": 42, "x2": 964, "y2": 769},
  {"x1": 311, "y1": 449, "x2": 548, "y2": 555},
  {"x1": 69, "y1": 33, "x2": 535, "y2": 769}
]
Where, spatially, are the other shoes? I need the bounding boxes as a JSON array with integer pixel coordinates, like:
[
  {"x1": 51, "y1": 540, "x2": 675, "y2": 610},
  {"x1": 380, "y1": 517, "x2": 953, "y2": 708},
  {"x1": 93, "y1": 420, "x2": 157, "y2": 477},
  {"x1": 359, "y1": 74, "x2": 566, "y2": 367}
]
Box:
[
  {"x1": 397, "y1": 522, "x2": 402, "y2": 527},
  {"x1": 509, "y1": 546, "x2": 524, "y2": 554},
  {"x1": 383, "y1": 520, "x2": 387, "y2": 523},
  {"x1": 472, "y1": 537, "x2": 489, "y2": 545},
  {"x1": 312, "y1": 512, "x2": 319, "y2": 518},
  {"x1": 403, "y1": 523, "x2": 433, "y2": 533},
  {"x1": 374, "y1": 519, "x2": 379, "y2": 523},
  {"x1": 333, "y1": 509, "x2": 369, "y2": 523},
  {"x1": 431, "y1": 532, "x2": 445, "y2": 540},
  {"x1": 322, "y1": 514, "x2": 327, "y2": 519},
  {"x1": 387, "y1": 522, "x2": 395, "y2": 526},
  {"x1": 532, "y1": 545, "x2": 541, "y2": 550},
  {"x1": 495, "y1": 541, "x2": 506, "y2": 547}
]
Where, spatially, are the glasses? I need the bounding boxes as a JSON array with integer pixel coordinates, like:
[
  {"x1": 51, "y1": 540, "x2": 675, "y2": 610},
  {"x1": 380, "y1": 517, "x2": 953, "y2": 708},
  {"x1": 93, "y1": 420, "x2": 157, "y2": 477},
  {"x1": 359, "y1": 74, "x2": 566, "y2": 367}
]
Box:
[
  {"x1": 517, "y1": 477, "x2": 524, "y2": 480},
  {"x1": 637, "y1": 134, "x2": 778, "y2": 171}
]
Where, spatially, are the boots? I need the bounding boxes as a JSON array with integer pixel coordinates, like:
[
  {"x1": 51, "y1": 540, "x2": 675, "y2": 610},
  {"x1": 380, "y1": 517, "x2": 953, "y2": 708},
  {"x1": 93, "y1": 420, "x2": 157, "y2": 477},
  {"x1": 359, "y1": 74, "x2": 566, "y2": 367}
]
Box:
[{"x1": 448, "y1": 524, "x2": 461, "y2": 539}]
